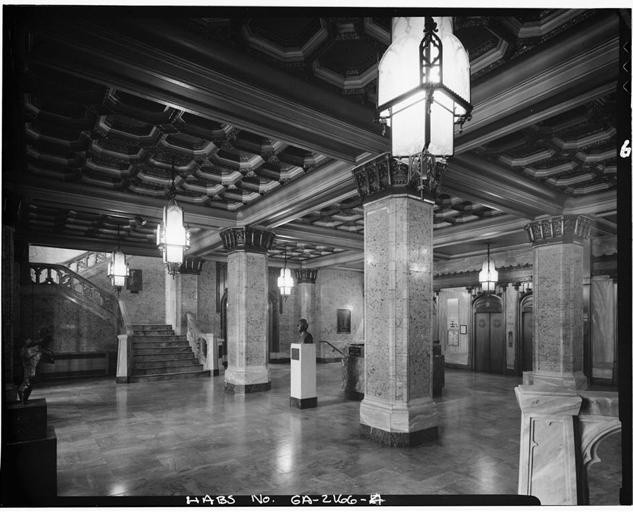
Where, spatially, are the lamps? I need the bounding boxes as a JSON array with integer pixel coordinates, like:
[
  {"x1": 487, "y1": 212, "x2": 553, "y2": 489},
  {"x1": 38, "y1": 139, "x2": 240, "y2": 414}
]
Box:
[
  {"x1": 278, "y1": 245, "x2": 295, "y2": 297},
  {"x1": 156, "y1": 160, "x2": 190, "y2": 278},
  {"x1": 374, "y1": 15, "x2": 471, "y2": 165},
  {"x1": 478, "y1": 242, "x2": 500, "y2": 291},
  {"x1": 108, "y1": 223, "x2": 130, "y2": 288}
]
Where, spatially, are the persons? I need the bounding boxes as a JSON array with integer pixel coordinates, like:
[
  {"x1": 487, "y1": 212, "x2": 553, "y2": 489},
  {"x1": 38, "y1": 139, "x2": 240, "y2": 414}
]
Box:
[{"x1": 293, "y1": 318, "x2": 313, "y2": 344}]
